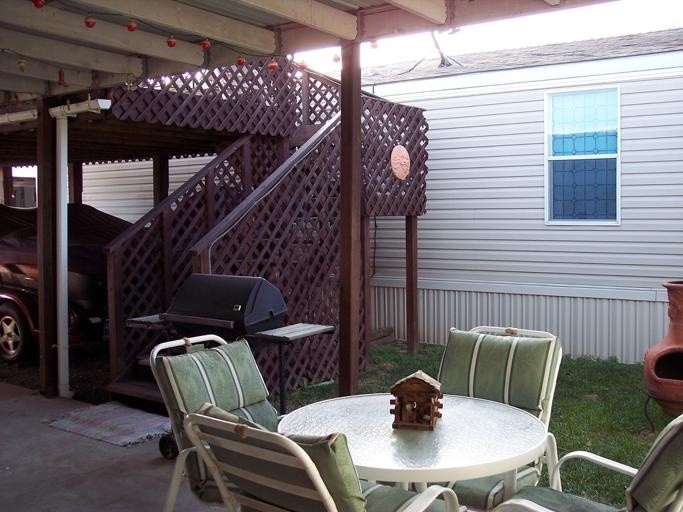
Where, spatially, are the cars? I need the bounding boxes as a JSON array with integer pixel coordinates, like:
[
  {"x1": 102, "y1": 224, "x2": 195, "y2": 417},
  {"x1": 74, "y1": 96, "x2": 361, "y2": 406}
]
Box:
[{"x1": 0, "y1": 202, "x2": 149, "y2": 365}]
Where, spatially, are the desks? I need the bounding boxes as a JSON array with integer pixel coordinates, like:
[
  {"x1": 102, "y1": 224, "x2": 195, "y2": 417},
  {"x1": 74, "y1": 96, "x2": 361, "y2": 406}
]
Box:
[{"x1": 125, "y1": 312, "x2": 335, "y2": 415}]
